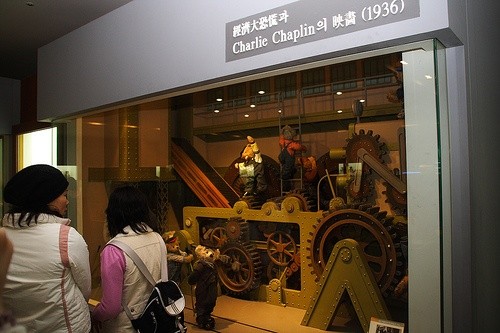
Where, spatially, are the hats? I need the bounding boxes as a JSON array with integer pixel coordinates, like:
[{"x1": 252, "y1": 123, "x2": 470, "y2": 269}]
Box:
[{"x1": 2, "y1": 164, "x2": 69, "y2": 205}]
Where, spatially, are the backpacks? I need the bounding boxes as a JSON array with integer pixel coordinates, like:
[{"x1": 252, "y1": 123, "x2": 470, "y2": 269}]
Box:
[{"x1": 99, "y1": 233, "x2": 187, "y2": 333}]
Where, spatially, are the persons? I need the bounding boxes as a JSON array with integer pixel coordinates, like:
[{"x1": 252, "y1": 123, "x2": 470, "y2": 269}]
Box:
[
  {"x1": 161, "y1": 230, "x2": 221, "y2": 329},
  {"x1": 235, "y1": 125, "x2": 311, "y2": 197},
  {"x1": 0, "y1": 164, "x2": 92, "y2": 333},
  {"x1": 88, "y1": 184, "x2": 168, "y2": 333}
]
